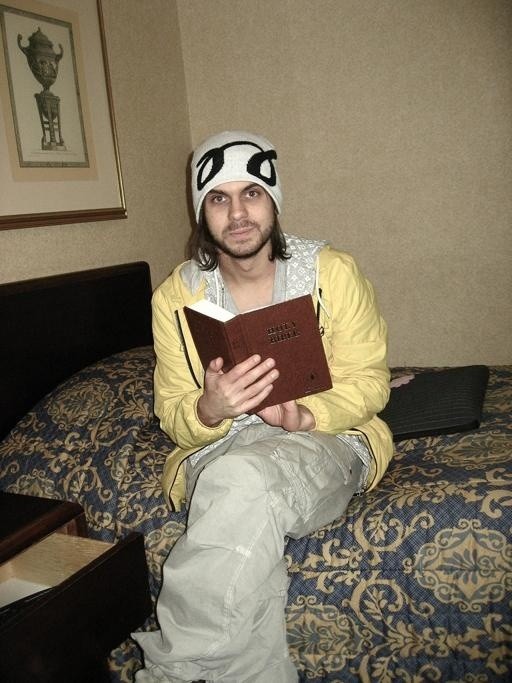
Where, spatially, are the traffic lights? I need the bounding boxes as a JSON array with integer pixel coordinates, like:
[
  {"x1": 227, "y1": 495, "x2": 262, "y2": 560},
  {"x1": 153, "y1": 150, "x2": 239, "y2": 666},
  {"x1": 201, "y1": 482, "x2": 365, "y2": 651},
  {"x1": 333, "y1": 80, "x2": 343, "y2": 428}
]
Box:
[{"x1": 377, "y1": 364, "x2": 489, "y2": 441}]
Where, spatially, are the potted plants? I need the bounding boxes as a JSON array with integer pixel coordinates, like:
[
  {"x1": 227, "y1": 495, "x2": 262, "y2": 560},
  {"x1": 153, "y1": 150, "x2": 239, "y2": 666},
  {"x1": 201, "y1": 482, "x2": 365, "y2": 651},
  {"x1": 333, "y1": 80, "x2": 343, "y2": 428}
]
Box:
[{"x1": 0, "y1": 0, "x2": 131, "y2": 230}]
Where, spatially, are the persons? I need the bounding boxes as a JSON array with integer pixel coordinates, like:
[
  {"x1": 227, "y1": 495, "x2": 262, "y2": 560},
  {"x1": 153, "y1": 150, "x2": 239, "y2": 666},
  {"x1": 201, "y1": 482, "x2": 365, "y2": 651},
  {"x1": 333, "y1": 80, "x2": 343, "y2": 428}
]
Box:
[{"x1": 131, "y1": 129, "x2": 396, "y2": 683}]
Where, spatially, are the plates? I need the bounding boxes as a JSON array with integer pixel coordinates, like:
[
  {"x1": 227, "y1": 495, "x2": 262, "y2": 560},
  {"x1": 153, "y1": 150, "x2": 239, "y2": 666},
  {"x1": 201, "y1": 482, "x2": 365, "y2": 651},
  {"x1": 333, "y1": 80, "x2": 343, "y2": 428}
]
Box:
[{"x1": 0, "y1": 487, "x2": 156, "y2": 683}]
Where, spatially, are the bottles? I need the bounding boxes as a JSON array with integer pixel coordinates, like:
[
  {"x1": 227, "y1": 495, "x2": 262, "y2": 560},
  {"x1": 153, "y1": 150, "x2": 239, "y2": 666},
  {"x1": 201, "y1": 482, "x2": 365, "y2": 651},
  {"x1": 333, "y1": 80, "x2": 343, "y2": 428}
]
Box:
[{"x1": 190, "y1": 128, "x2": 283, "y2": 225}]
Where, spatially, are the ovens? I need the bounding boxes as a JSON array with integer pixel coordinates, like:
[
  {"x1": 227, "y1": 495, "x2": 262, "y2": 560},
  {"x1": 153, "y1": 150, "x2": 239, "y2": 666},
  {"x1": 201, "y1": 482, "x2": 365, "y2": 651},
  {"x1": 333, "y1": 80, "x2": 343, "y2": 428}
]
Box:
[{"x1": 0, "y1": 260, "x2": 511, "y2": 683}]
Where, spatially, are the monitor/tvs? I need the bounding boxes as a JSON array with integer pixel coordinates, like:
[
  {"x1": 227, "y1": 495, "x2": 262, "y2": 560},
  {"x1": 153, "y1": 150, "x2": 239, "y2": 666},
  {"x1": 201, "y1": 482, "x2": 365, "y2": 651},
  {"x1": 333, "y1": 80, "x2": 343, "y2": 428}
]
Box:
[{"x1": 183, "y1": 293, "x2": 334, "y2": 416}]
